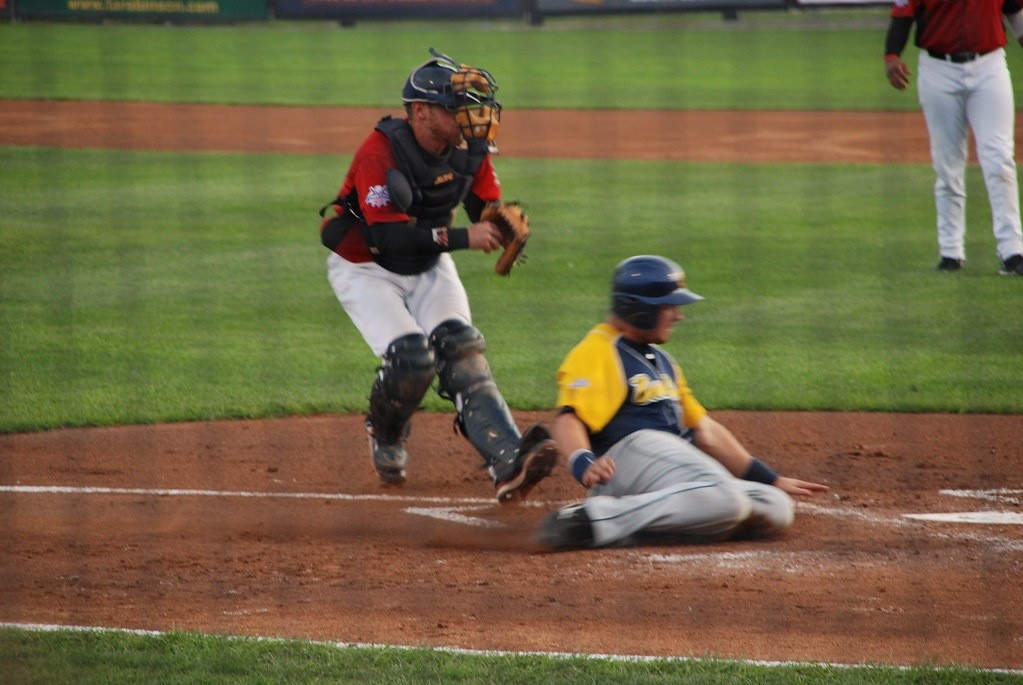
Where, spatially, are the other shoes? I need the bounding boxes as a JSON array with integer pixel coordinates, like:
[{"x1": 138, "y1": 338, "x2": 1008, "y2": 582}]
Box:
[
  {"x1": 938, "y1": 258, "x2": 960, "y2": 273},
  {"x1": 999, "y1": 252, "x2": 1023, "y2": 277}
]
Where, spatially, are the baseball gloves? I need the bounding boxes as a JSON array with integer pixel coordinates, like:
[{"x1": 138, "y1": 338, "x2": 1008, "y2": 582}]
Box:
[{"x1": 477, "y1": 199, "x2": 533, "y2": 280}]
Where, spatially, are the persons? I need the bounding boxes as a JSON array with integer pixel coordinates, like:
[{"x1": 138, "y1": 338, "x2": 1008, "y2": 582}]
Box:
[
  {"x1": 538, "y1": 257, "x2": 831, "y2": 541},
  {"x1": 320, "y1": 47, "x2": 560, "y2": 505},
  {"x1": 883, "y1": 0, "x2": 1023, "y2": 280}
]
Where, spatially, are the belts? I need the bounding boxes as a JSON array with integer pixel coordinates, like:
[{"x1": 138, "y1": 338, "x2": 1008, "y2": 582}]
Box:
[{"x1": 927, "y1": 49, "x2": 984, "y2": 63}]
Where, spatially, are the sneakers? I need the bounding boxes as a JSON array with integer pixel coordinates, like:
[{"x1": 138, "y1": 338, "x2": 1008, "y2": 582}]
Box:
[
  {"x1": 496, "y1": 437, "x2": 559, "y2": 510},
  {"x1": 368, "y1": 425, "x2": 408, "y2": 485},
  {"x1": 532, "y1": 499, "x2": 593, "y2": 551}
]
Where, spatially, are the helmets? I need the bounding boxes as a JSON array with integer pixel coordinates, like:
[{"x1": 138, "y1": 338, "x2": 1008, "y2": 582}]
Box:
[
  {"x1": 400, "y1": 46, "x2": 463, "y2": 114},
  {"x1": 611, "y1": 253, "x2": 705, "y2": 304}
]
